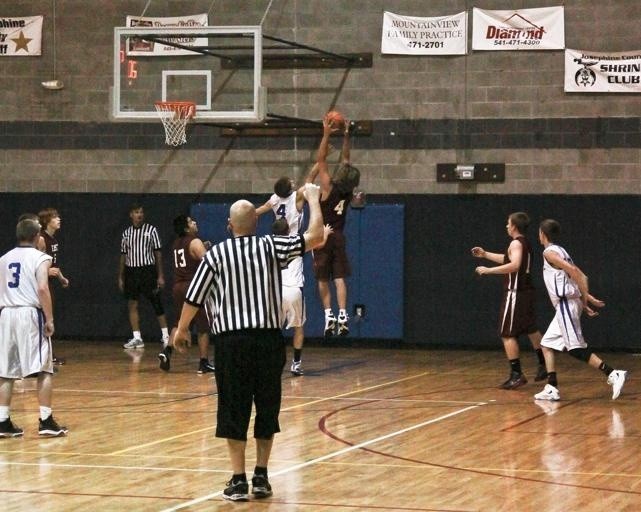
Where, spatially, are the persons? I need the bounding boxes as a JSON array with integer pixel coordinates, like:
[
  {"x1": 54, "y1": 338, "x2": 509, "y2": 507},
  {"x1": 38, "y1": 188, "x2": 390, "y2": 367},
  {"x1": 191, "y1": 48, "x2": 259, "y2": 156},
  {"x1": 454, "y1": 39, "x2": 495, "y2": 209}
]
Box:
[
  {"x1": 534, "y1": 219, "x2": 629, "y2": 401},
  {"x1": 272, "y1": 218, "x2": 334, "y2": 375},
  {"x1": 38, "y1": 207, "x2": 69, "y2": 365},
  {"x1": 1, "y1": 214, "x2": 68, "y2": 437},
  {"x1": 157, "y1": 214, "x2": 215, "y2": 374},
  {"x1": 312, "y1": 117, "x2": 360, "y2": 341},
  {"x1": 117, "y1": 202, "x2": 169, "y2": 349},
  {"x1": 470, "y1": 212, "x2": 547, "y2": 389},
  {"x1": 18, "y1": 213, "x2": 60, "y2": 278},
  {"x1": 227, "y1": 145, "x2": 335, "y2": 237},
  {"x1": 172, "y1": 183, "x2": 325, "y2": 501}
]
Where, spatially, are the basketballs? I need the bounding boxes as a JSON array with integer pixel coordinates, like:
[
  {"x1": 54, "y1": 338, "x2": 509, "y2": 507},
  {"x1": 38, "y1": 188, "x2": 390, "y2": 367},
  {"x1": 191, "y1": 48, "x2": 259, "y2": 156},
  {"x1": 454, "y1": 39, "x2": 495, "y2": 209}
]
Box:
[{"x1": 326, "y1": 112, "x2": 344, "y2": 131}]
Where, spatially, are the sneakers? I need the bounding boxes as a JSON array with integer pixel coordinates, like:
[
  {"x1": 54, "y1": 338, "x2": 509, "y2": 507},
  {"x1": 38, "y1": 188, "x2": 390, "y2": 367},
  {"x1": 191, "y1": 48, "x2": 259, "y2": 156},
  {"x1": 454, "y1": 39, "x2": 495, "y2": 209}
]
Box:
[
  {"x1": 53, "y1": 356, "x2": 65, "y2": 365},
  {"x1": 222, "y1": 479, "x2": 249, "y2": 501},
  {"x1": 123, "y1": 334, "x2": 215, "y2": 376},
  {"x1": 324, "y1": 315, "x2": 338, "y2": 338},
  {"x1": 252, "y1": 476, "x2": 273, "y2": 497},
  {"x1": 337, "y1": 315, "x2": 350, "y2": 337},
  {"x1": 1, "y1": 415, "x2": 25, "y2": 439},
  {"x1": 39, "y1": 415, "x2": 70, "y2": 437},
  {"x1": 290, "y1": 361, "x2": 304, "y2": 376},
  {"x1": 499, "y1": 368, "x2": 630, "y2": 401}
]
